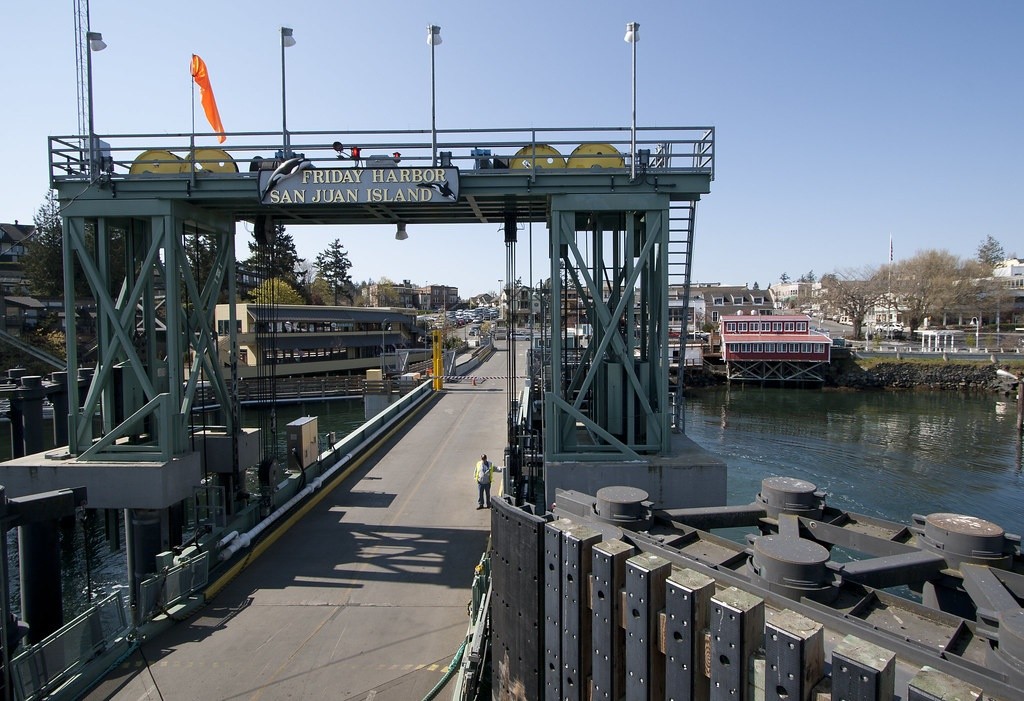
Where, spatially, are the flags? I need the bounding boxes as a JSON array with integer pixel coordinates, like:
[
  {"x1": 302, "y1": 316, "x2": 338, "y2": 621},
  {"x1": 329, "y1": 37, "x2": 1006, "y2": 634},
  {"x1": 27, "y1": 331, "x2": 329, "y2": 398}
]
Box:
[
  {"x1": 891, "y1": 239, "x2": 893, "y2": 261},
  {"x1": 190, "y1": 54, "x2": 227, "y2": 143}
]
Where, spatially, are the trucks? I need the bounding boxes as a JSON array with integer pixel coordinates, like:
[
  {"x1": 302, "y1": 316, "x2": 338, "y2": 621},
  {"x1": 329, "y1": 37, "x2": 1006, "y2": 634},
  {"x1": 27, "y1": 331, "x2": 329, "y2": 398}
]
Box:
[
  {"x1": 574, "y1": 323, "x2": 594, "y2": 336},
  {"x1": 839, "y1": 316, "x2": 867, "y2": 326}
]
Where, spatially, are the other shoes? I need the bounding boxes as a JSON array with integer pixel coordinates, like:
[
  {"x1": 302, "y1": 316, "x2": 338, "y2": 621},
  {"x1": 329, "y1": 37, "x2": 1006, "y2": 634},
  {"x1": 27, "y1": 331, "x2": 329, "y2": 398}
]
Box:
[
  {"x1": 477, "y1": 505, "x2": 483, "y2": 509},
  {"x1": 487, "y1": 506, "x2": 492, "y2": 509}
]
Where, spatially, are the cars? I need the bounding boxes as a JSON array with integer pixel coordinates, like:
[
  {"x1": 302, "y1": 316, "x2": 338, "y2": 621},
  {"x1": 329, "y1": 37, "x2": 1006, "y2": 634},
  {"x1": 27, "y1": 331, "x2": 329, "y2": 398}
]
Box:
[
  {"x1": 417, "y1": 306, "x2": 501, "y2": 332},
  {"x1": 494, "y1": 329, "x2": 515, "y2": 341},
  {"x1": 875, "y1": 323, "x2": 903, "y2": 333},
  {"x1": 566, "y1": 328, "x2": 575, "y2": 337},
  {"x1": 506, "y1": 333, "x2": 534, "y2": 341},
  {"x1": 469, "y1": 326, "x2": 481, "y2": 336},
  {"x1": 803, "y1": 308, "x2": 840, "y2": 321}
]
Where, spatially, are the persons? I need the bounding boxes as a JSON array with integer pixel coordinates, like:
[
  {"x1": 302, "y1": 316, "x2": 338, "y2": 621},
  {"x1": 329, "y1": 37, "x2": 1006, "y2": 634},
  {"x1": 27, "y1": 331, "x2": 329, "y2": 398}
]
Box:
[{"x1": 473, "y1": 454, "x2": 503, "y2": 510}]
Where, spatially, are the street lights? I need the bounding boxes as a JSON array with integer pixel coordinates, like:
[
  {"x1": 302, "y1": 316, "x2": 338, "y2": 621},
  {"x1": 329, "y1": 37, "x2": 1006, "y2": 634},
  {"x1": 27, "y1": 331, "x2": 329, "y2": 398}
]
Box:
[
  {"x1": 85, "y1": 31, "x2": 108, "y2": 180},
  {"x1": 625, "y1": 20, "x2": 641, "y2": 182},
  {"x1": 970, "y1": 316, "x2": 980, "y2": 351},
  {"x1": 381, "y1": 318, "x2": 392, "y2": 373},
  {"x1": 498, "y1": 279, "x2": 504, "y2": 296},
  {"x1": 427, "y1": 23, "x2": 442, "y2": 167},
  {"x1": 279, "y1": 24, "x2": 298, "y2": 160},
  {"x1": 693, "y1": 300, "x2": 696, "y2": 341}
]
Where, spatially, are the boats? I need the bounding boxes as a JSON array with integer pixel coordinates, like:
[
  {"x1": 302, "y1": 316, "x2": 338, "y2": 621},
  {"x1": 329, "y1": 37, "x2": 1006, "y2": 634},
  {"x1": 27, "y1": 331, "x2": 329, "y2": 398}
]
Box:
[{"x1": 213, "y1": 304, "x2": 446, "y2": 380}]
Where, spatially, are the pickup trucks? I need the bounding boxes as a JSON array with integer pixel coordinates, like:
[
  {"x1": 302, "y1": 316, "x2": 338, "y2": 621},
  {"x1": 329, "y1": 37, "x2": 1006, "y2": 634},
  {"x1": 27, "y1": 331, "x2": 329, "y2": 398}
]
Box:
[{"x1": 688, "y1": 330, "x2": 711, "y2": 338}]
